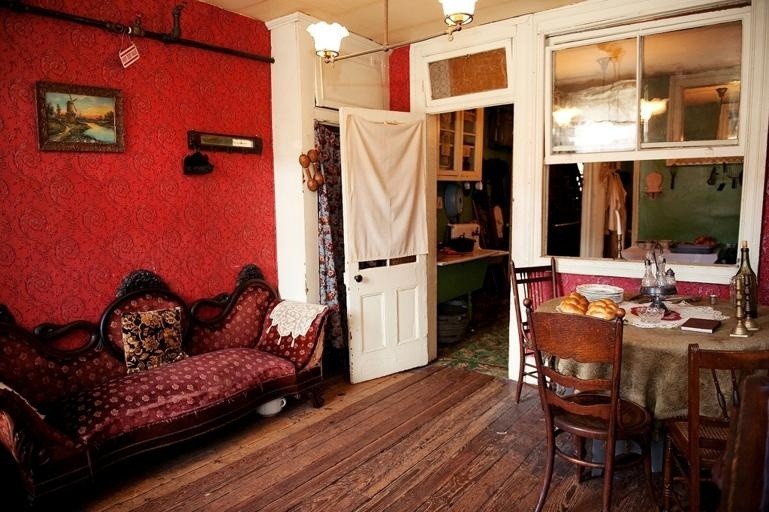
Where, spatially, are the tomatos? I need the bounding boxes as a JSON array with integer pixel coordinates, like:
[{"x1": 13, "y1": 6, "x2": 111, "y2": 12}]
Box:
[{"x1": 693, "y1": 235, "x2": 716, "y2": 246}]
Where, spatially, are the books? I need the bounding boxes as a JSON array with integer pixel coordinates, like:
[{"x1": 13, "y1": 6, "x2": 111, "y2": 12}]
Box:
[{"x1": 680, "y1": 318, "x2": 721, "y2": 334}]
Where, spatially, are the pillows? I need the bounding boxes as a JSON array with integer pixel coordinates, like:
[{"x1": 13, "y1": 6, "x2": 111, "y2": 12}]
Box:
[{"x1": 120, "y1": 306, "x2": 190, "y2": 374}]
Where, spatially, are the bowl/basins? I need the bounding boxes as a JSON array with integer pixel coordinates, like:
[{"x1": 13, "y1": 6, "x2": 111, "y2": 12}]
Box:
[
  {"x1": 451, "y1": 237, "x2": 475, "y2": 252},
  {"x1": 637, "y1": 308, "x2": 665, "y2": 324},
  {"x1": 443, "y1": 182, "x2": 464, "y2": 218},
  {"x1": 633, "y1": 238, "x2": 677, "y2": 249},
  {"x1": 437, "y1": 305, "x2": 469, "y2": 346}
]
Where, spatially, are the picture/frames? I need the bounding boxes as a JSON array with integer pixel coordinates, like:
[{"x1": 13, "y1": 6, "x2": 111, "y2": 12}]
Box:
[{"x1": 34, "y1": 79, "x2": 126, "y2": 154}]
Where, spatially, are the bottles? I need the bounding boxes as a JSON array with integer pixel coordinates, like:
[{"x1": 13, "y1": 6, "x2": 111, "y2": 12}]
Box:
[
  {"x1": 735, "y1": 241, "x2": 759, "y2": 319},
  {"x1": 641, "y1": 256, "x2": 676, "y2": 287}
]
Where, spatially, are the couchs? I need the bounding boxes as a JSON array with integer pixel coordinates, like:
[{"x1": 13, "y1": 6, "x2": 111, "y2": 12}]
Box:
[{"x1": 0, "y1": 261, "x2": 335, "y2": 512}]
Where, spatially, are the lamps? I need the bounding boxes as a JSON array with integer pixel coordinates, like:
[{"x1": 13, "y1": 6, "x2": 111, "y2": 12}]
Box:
[{"x1": 306, "y1": 0, "x2": 478, "y2": 68}]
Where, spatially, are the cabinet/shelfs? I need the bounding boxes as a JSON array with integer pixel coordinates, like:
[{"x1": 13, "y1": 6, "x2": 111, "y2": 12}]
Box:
[{"x1": 436, "y1": 107, "x2": 485, "y2": 182}]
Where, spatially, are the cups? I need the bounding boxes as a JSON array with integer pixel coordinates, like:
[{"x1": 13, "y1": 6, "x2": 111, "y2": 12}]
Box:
[
  {"x1": 256, "y1": 397, "x2": 286, "y2": 418},
  {"x1": 709, "y1": 294, "x2": 719, "y2": 305}
]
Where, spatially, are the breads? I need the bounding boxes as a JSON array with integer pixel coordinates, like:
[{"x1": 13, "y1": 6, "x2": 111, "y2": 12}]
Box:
[{"x1": 561, "y1": 291, "x2": 625, "y2": 319}]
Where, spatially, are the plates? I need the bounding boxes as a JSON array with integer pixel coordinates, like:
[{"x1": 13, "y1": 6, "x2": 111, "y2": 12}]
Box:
[{"x1": 575, "y1": 283, "x2": 624, "y2": 306}]
